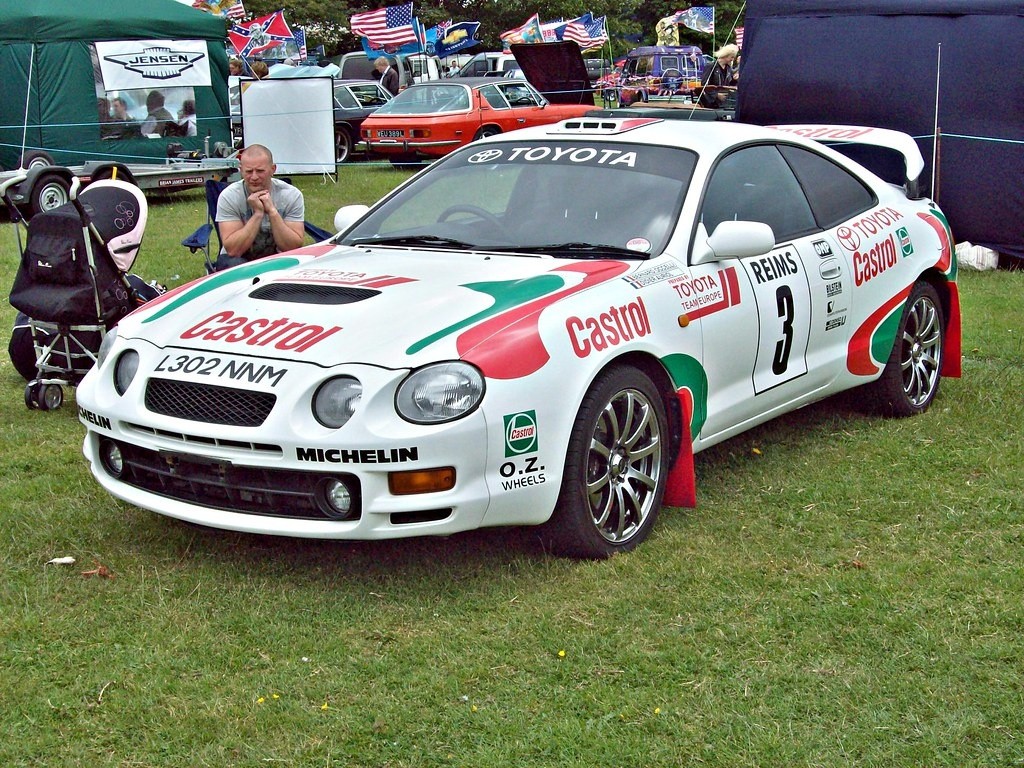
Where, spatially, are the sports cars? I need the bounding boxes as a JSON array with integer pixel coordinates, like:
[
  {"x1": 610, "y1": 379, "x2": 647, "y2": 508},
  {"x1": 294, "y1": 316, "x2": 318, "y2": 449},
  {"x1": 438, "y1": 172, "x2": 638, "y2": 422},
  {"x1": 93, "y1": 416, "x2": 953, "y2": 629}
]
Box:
[{"x1": 72, "y1": 106, "x2": 963, "y2": 562}]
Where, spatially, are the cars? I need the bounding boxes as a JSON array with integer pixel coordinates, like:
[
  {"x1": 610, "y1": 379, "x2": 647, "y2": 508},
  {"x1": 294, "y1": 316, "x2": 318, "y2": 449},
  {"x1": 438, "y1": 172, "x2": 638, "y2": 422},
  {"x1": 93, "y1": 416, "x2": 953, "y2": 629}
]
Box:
[{"x1": 227, "y1": 45, "x2": 718, "y2": 171}]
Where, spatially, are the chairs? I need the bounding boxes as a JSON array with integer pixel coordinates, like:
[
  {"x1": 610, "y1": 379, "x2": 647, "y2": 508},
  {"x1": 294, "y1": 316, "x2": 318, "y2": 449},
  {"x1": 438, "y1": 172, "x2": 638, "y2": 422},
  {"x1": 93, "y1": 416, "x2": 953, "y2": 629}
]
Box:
[
  {"x1": 181, "y1": 175, "x2": 331, "y2": 277},
  {"x1": 517, "y1": 170, "x2": 612, "y2": 244},
  {"x1": 690, "y1": 85, "x2": 720, "y2": 109},
  {"x1": 163, "y1": 120, "x2": 189, "y2": 138},
  {"x1": 486, "y1": 93, "x2": 507, "y2": 108},
  {"x1": 437, "y1": 94, "x2": 456, "y2": 110},
  {"x1": 338, "y1": 90, "x2": 353, "y2": 108},
  {"x1": 707, "y1": 178, "x2": 773, "y2": 229}
]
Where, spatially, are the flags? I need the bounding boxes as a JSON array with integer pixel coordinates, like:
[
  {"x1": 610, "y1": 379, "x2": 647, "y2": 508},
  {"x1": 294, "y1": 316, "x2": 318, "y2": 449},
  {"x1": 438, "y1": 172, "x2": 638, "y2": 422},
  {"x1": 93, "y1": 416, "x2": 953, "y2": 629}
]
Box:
[
  {"x1": 226, "y1": 1, "x2": 307, "y2": 61},
  {"x1": 673, "y1": 7, "x2": 715, "y2": 35},
  {"x1": 735, "y1": 28, "x2": 744, "y2": 49},
  {"x1": 349, "y1": 2, "x2": 482, "y2": 62},
  {"x1": 500, "y1": 12, "x2": 609, "y2": 55}
]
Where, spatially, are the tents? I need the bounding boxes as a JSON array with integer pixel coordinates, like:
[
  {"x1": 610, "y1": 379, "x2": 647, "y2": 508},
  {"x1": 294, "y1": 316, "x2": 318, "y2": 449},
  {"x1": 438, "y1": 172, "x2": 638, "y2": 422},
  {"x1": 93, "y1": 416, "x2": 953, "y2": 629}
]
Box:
[
  {"x1": 0, "y1": 0, "x2": 235, "y2": 193},
  {"x1": 741, "y1": 0, "x2": 1024, "y2": 265}
]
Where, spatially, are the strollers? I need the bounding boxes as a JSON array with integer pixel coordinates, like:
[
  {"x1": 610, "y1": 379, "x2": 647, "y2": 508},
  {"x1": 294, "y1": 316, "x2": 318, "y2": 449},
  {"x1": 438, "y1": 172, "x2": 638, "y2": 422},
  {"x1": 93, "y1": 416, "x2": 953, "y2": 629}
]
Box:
[{"x1": 0, "y1": 174, "x2": 169, "y2": 412}]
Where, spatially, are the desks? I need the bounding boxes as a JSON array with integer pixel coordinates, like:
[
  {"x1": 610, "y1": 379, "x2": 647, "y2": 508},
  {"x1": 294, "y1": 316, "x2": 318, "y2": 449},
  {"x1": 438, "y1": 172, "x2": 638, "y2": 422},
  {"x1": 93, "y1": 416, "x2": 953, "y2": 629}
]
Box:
[
  {"x1": 632, "y1": 103, "x2": 704, "y2": 109},
  {"x1": 618, "y1": 87, "x2": 648, "y2": 109}
]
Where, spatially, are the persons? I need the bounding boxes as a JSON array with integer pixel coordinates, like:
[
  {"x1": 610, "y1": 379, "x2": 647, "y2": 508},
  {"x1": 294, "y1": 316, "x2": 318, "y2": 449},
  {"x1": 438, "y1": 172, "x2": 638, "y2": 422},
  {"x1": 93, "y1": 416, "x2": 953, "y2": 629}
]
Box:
[
  {"x1": 374, "y1": 56, "x2": 399, "y2": 96},
  {"x1": 97, "y1": 90, "x2": 197, "y2": 140},
  {"x1": 442, "y1": 60, "x2": 461, "y2": 79},
  {"x1": 213, "y1": 144, "x2": 305, "y2": 271},
  {"x1": 229, "y1": 61, "x2": 250, "y2": 77},
  {"x1": 249, "y1": 61, "x2": 271, "y2": 79},
  {"x1": 701, "y1": 44, "x2": 741, "y2": 110}
]
